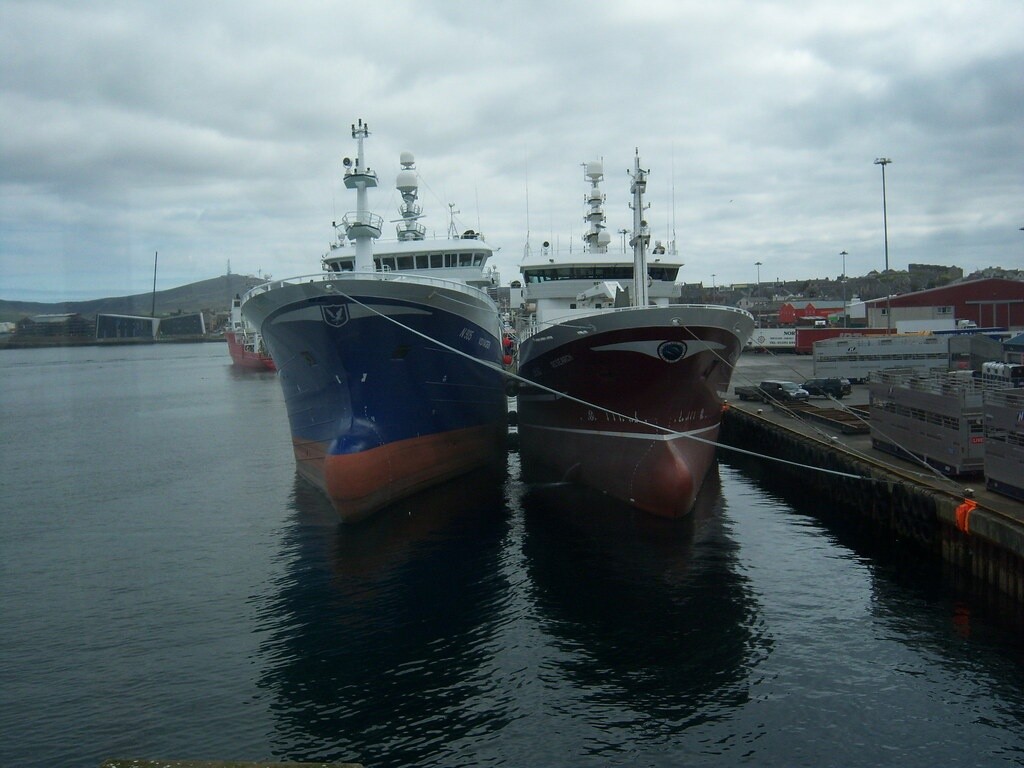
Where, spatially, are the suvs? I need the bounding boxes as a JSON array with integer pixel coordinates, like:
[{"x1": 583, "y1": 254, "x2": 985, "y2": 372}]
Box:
[
  {"x1": 798, "y1": 376, "x2": 853, "y2": 400},
  {"x1": 759, "y1": 379, "x2": 810, "y2": 405}
]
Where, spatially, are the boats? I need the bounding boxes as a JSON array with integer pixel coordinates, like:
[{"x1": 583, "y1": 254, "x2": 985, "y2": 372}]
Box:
[
  {"x1": 224, "y1": 266, "x2": 276, "y2": 371},
  {"x1": 241, "y1": 117, "x2": 510, "y2": 527},
  {"x1": 514, "y1": 146, "x2": 760, "y2": 523}
]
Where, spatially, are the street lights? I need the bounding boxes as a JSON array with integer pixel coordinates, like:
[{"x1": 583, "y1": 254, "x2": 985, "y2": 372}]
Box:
[
  {"x1": 754, "y1": 261, "x2": 763, "y2": 283},
  {"x1": 839, "y1": 250, "x2": 849, "y2": 328},
  {"x1": 873, "y1": 156, "x2": 893, "y2": 335},
  {"x1": 711, "y1": 274, "x2": 716, "y2": 286}
]
